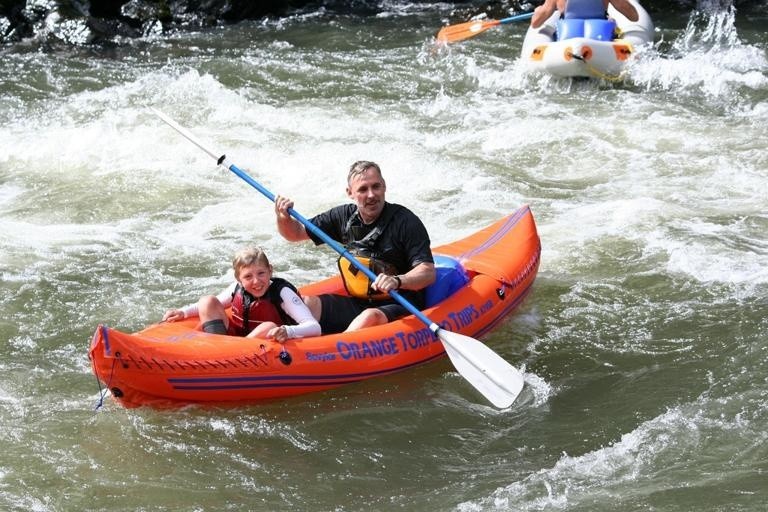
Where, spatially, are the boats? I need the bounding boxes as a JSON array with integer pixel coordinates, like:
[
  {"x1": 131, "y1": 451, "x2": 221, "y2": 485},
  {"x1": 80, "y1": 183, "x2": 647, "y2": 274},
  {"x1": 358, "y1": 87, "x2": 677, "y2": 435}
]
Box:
[
  {"x1": 88, "y1": 204, "x2": 541, "y2": 410},
  {"x1": 520, "y1": 0, "x2": 654, "y2": 80}
]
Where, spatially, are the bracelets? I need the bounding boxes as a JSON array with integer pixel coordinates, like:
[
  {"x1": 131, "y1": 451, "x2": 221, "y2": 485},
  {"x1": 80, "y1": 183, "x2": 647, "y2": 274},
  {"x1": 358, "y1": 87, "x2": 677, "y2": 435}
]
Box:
[{"x1": 394, "y1": 276, "x2": 401, "y2": 290}]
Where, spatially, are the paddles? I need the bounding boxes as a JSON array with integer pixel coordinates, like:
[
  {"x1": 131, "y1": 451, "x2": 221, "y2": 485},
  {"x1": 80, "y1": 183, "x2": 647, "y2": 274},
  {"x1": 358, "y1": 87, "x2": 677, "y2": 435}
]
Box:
[
  {"x1": 149, "y1": 106, "x2": 524, "y2": 409},
  {"x1": 437, "y1": 12, "x2": 533, "y2": 43}
]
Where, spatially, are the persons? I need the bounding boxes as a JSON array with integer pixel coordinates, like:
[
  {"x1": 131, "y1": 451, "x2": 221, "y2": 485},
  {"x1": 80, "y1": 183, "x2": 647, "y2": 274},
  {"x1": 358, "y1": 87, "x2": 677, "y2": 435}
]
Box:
[
  {"x1": 274, "y1": 161, "x2": 436, "y2": 332},
  {"x1": 162, "y1": 247, "x2": 321, "y2": 343},
  {"x1": 532, "y1": 0, "x2": 639, "y2": 41}
]
